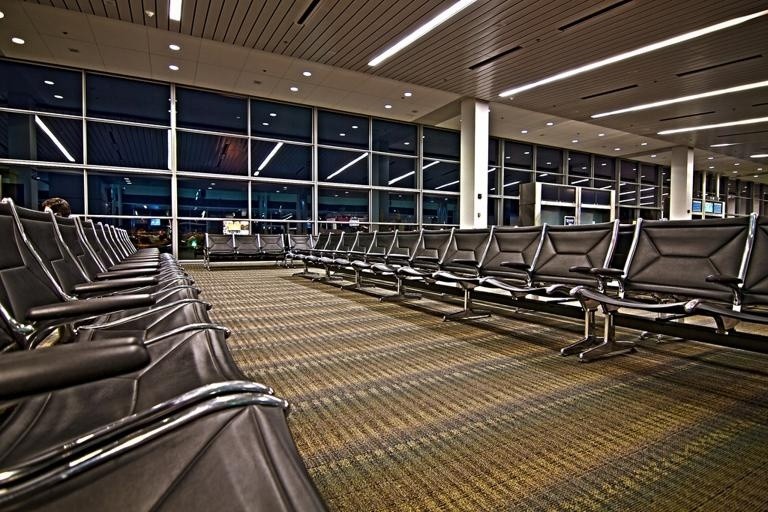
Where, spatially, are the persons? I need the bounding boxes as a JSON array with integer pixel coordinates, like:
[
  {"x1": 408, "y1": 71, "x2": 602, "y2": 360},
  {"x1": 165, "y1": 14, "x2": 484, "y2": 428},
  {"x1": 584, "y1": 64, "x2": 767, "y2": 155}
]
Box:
[{"x1": 40, "y1": 197, "x2": 73, "y2": 219}]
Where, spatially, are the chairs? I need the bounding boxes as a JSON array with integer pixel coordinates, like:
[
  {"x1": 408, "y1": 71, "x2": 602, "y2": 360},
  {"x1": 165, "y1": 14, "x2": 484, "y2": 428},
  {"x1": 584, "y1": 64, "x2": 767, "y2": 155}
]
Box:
[
  {"x1": 286, "y1": 207, "x2": 766, "y2": 364},
  {"x1": 0, "y1": 197, "x2": 329, "y2": 512}
]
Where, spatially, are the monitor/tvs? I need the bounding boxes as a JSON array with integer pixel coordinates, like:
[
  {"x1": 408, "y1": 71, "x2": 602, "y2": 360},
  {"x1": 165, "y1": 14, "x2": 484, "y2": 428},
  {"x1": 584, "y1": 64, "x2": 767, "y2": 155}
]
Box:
[
  {"x1": 692, "y1": 200, "x2": 702, "y2": 212},
  {"x1": 705, "y1": 201, "x2": 714, "y2": 213},
  {"x1": 714, "y1": 202, "x2": 722, "y2": 214},
  {"x1": 149, "y1": 219, "x2": 161, "y2": 227}
]
